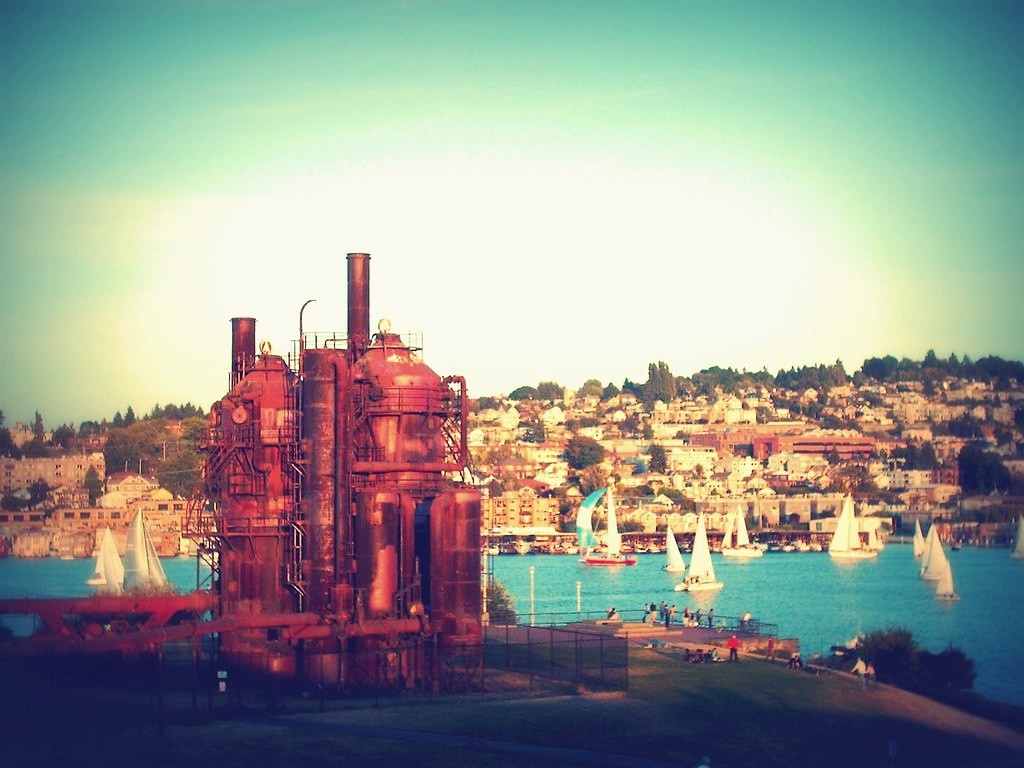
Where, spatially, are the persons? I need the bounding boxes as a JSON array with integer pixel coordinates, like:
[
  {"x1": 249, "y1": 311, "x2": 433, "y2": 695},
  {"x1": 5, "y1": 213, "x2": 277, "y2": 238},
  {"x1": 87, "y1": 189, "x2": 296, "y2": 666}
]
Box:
[
  {"x1": 607, "y1": 576, "x2": 752, "y2": 629},
  {"x1": 685, "y1": 635, "x2": 878, "y2": 689}
]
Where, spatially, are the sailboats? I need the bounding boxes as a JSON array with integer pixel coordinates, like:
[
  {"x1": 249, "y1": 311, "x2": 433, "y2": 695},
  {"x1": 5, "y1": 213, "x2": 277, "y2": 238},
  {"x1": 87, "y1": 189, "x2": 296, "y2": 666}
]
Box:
[
  {"x1": 1008, "y1": 513, "x2": 1024, "y2": 561},
  {"x1": 913, "y1": 516, "x2": 960, "y2": 601},
  {"x1": 722, "y1": 507, "x2": 765, "y2": 557},
  {"x1": 85, "y1": 506, "x2": 172, "y2": 599},
  {"x1": 575, "y1": 486, "x2": 641, "y2": 567},
  {"x1": 673, "y1": 513, "x2": 724, "y2": 593},
  {"x1": 827, "y1": 490, "x2": 885, "y2": 558},
  {"x1": 661, "y1": 525, "x2": 688, "y2": 574}
]
